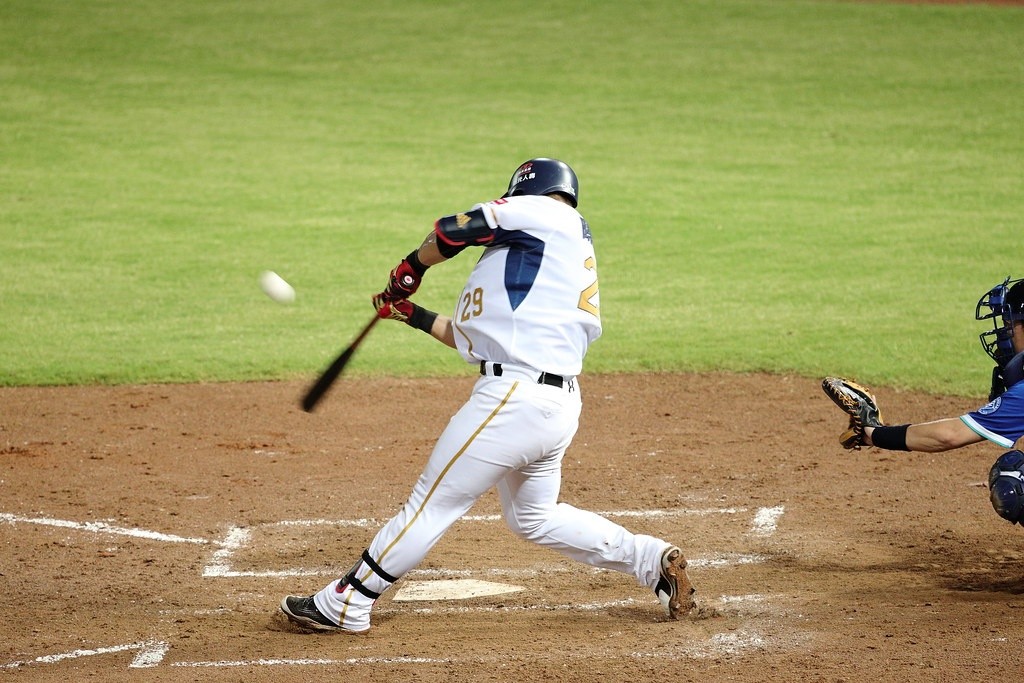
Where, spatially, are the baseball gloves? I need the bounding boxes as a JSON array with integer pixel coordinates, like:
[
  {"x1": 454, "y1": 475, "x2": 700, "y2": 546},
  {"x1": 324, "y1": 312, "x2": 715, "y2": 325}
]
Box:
[{"x1": 821, "y1": 376, "x2": 884, "y2": 450}]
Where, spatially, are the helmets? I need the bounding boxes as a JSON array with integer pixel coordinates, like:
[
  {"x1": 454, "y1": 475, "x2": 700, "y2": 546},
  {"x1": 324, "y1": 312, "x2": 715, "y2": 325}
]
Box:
[{"x1": 501, "y1": 158, "x2": 578, "y2": 209}]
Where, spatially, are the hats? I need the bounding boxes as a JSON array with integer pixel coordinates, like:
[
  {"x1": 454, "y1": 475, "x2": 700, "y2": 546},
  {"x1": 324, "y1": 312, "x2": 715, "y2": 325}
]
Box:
[{"x1": 1002, "y1": 280, "x2": 1024, "y2": 320}]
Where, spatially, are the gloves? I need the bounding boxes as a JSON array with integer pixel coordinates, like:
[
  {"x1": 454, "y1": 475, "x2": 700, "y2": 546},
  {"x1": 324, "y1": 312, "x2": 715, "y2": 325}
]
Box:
[
  {"x1": 384, "y1": 249, "x2": 430, "y2": 300},
  {"x1": 372, "y1": 293, "x2": 425, "y2": 329}
]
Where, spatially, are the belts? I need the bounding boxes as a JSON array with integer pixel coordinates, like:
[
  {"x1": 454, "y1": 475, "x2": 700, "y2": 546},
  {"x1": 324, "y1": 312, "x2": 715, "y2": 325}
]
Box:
[{"x1": 480, "y1": 360, "x2": 563, "y2": 389}]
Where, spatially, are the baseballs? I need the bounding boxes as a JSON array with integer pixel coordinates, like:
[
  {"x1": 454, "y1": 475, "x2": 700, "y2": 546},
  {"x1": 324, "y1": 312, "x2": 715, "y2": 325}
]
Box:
[{"x1": 256, "y1": 270, "x2": 300, "y2": 305}]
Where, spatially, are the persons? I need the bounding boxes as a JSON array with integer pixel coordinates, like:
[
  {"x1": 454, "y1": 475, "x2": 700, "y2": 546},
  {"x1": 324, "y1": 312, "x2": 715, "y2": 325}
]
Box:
[
  {"x1": 281, "y1": 158, "x2": 697, "y2": 634},
  {"x1": 821, "y1": 278, "x2": 1024, "y2": 530}
]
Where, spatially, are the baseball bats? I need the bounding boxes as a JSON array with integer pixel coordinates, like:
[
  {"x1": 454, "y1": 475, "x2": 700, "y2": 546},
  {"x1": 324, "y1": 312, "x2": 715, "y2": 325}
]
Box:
[{"x1": 298, "y1": 312, "x2": 382, "y2": 413}]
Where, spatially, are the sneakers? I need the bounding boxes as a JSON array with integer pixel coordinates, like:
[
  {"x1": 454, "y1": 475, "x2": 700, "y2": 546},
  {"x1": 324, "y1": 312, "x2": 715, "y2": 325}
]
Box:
[
  {"x1": 655, "y1": 545, "x2": 697, "y2": 620},
  {"x1": 281, "y1": 595, "x2": 369, "y2": 635}
]
